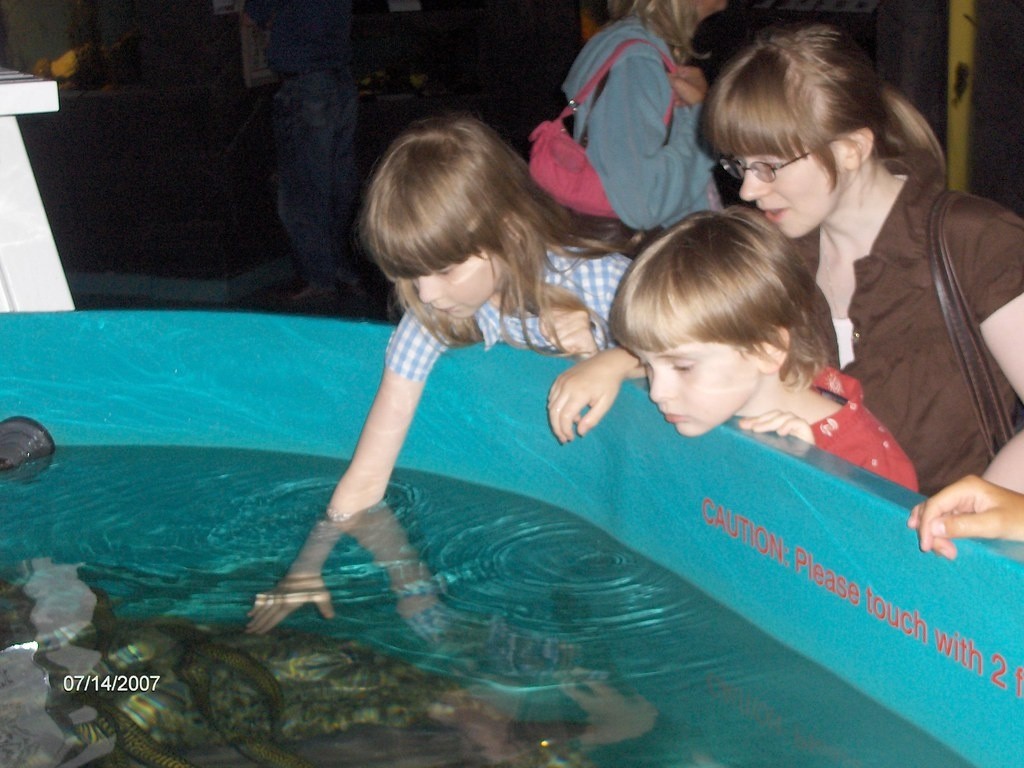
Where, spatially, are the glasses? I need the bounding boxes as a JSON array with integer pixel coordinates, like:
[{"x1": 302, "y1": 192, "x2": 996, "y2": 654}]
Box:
[{"x1": 720, "y1": 152, "x2": 815, "y2": 180}]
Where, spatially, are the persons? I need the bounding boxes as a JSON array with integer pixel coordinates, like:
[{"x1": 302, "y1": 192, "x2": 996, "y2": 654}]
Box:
[
  {"x1": 563, "y1": 0, "x2": 720, "y2": 232},
  {"x1": 547, "y1": 205, "x2": 917, "y2": 490},
  {"x1": 907, "y1": 475, "x2": 1024, "y2": 560},
  {"x1": 710, "y1": 26, "x2": 1024, "y2": 496},
  {"x1": 243, "y1": 1, "x2": 370, "y2": 299},
  {"x1": 327, "y1": 114, "x2": 637, "y2": 519}
]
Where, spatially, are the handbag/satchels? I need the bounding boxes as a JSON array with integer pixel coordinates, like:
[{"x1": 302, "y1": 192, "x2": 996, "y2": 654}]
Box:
[{"x1": 531, "y1": 38, "x2": 676, "y2": 219}]
